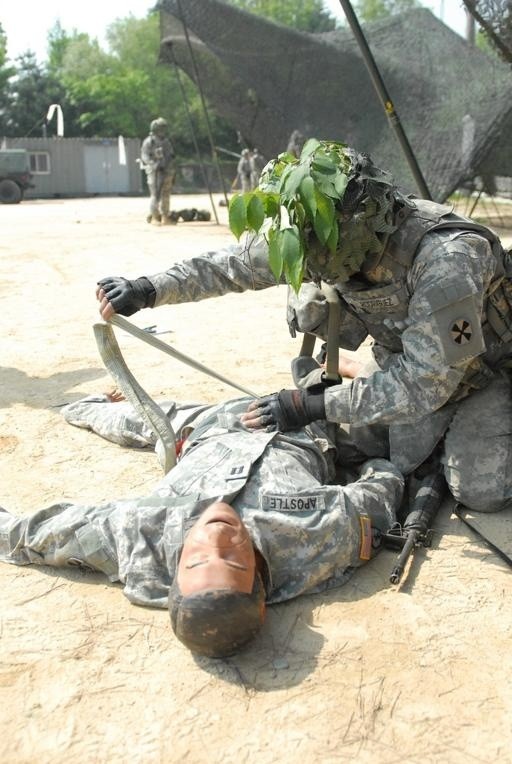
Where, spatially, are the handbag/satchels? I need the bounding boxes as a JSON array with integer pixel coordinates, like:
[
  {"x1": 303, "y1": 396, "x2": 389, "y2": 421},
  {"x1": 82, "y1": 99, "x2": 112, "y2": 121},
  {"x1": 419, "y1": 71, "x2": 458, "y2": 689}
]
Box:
[{"x1": 292, "y1": 357, "x2": 342, "y2": 446}]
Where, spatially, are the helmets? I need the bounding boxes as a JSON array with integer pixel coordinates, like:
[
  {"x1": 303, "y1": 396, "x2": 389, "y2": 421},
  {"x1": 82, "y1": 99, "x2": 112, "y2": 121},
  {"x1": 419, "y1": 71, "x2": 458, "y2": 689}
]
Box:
[{"x1": 148, "y1": 117, "x2": 168, "y2": 134}]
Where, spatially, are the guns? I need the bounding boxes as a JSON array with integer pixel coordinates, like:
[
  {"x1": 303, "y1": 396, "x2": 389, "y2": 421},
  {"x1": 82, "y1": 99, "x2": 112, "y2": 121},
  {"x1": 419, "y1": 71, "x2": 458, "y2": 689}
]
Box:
[{"x1": 379, "y1": 473, "x2": 447, "y2": 584}]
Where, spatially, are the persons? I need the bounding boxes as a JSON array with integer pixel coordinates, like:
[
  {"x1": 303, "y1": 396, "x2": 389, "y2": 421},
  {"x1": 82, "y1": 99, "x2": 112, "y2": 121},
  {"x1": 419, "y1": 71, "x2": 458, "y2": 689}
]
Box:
[
  {"x1": 141, "y1": 117, "x2": 177, "y2": 225},
  {"x1": 250, "y1": 146, "x2": 268, "y2": 191},
  {"x1": 235, "y1": 147, "x2": 251, "y2": 193},
  {"x1": 93, "y1": 137, "x2": 512, "y2": 514},
  {"x1": 286, "y1": 128, "x2": 303, "y2": 161},
  {"x1": 0, "y1": 354, "x2": 409, "y2": 662}
]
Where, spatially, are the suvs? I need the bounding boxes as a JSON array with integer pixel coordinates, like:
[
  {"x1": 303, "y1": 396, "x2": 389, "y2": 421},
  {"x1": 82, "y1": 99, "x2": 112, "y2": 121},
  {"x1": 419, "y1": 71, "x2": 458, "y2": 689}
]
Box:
[{"x1": 1, "y1": 148, "x2": 39, "y2": 205}]
